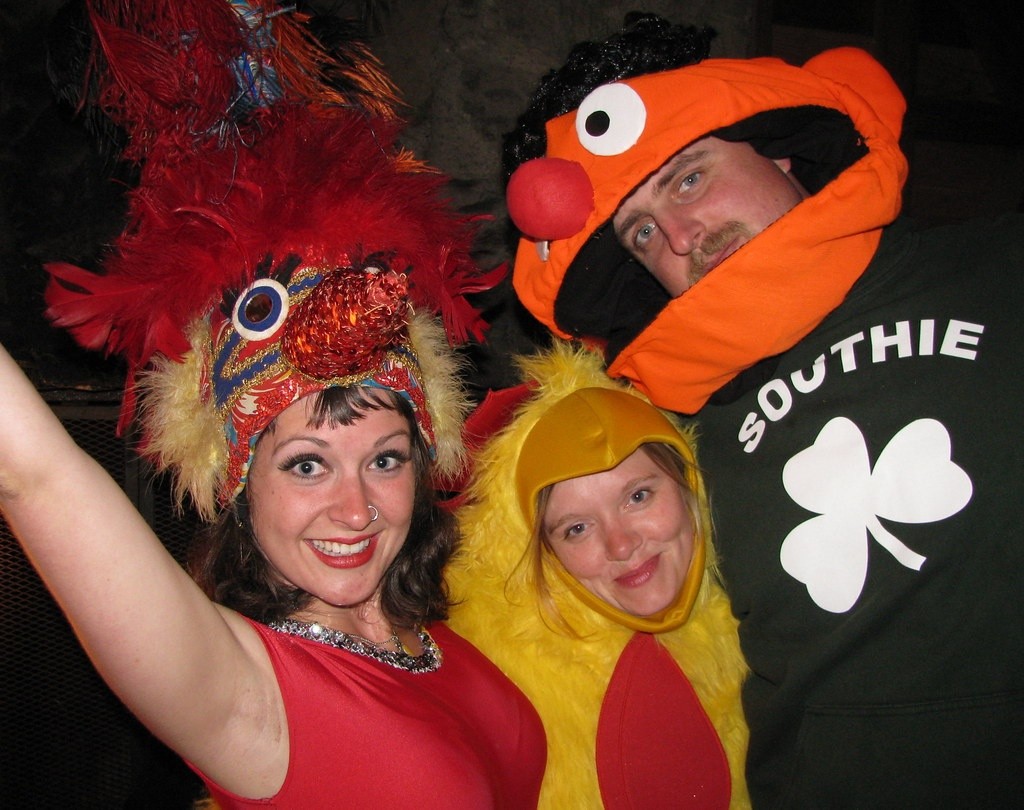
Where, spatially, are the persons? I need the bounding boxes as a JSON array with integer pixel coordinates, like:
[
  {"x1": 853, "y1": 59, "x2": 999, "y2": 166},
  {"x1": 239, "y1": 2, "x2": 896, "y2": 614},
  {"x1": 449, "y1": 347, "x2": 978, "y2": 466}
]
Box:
[
  {"x1": 0, "y1": 269, "x2": 548, "y2": 810},
  {"x1": 501, "y1": 14, "x2": 1024, "y2": 810},
  {"x1": 442, "y1": 343, "x2": 755, "y2": 809}
]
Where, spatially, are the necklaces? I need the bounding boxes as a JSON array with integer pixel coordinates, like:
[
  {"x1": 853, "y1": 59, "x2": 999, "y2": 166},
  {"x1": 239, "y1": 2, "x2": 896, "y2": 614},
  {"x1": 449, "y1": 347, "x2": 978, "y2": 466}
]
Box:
[{"x1": 316, "y1": 621, "x2": 415, "y2": 657}]
